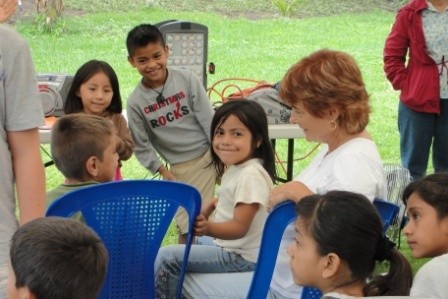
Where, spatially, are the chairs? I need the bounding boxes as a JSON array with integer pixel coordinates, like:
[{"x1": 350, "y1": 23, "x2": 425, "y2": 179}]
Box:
[
  {"x1": 246, "y1": 195, "x2": 400, "y2": 299},
  {"x1": 45, "y1": 180, "x2": 202, "y2": 299}
]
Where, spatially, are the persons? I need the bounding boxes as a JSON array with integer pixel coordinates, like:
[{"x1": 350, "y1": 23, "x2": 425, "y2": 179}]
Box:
[
  {"x1": 43, "y1": 113, "x2": 119, "y2": 222},
  {"x1": 384, "y1": 0, "x2": 448, "y2": 228},
  {"x1": 5, "y1": 218, "x2": 115, "y2": 299},
  {"x1": 285, "y1": 190, "x2": 414, "y2": 299},
  {"x1": 182, "y1": 47, "x2": 387, "y2": 299},
  {"x1": 154, "y1": 99, "x2": 277, "y2": 299},
  {"x1": 402, "y1": 172, "x2": 447, "y2": 299},
  {"x1": 0, "y1": 0, "x2": 48, "y2": 298},
  {"x1": 63, "y1": 59, "x2": 135, "y2": 181},
  {"x1": 126, "y1": 22, "x2": 224, "y2": 244}
]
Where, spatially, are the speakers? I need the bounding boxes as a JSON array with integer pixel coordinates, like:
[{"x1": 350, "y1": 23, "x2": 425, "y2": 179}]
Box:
[{"x1": 36, "y1": 73, "x2": 75, "y2": 118}]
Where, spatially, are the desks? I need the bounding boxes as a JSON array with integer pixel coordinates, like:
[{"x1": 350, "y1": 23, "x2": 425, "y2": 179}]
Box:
[{"x1": 39, "y1": 122, "x2": 305, "y2": 183}]
